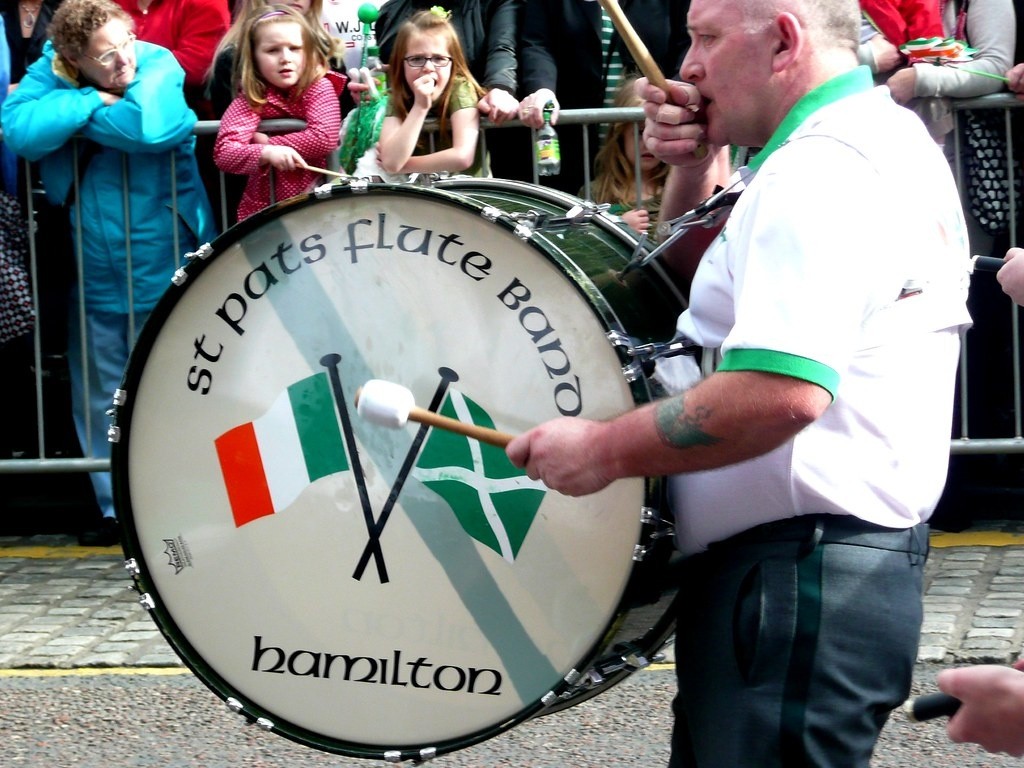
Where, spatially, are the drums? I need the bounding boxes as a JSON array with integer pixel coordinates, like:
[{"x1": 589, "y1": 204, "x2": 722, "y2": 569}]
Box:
[{"x1": 106, "y1": 175, "x2": 684, "y2": 760}]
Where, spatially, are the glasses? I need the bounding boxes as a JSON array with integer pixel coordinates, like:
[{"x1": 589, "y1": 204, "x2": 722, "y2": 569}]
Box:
[
  {"x1": 402, "y1": 54, "x2": 455, "y2": 67},
  {"x1": 82, "y1": 31, "x2": 135, "y2": 65}
]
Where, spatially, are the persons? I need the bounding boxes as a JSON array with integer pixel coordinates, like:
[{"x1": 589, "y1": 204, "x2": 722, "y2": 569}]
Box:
[
  {"x1": 935, "y1": 657, "x2": 1024, "y2": 757},
  {"x1": 0, "y1": 0, "x2": 1023, "y2": 547},
  {"x1": 505, "y1": 2, "x2": 973, "y2": 768}
]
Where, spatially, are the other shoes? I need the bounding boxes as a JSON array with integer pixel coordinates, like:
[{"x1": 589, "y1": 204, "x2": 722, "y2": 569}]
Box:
[{"x1": 80, "y1": 521, "x2": 119, "y2": 545}]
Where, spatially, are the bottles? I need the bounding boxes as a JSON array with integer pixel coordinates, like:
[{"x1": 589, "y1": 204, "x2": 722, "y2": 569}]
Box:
[
  {"x1": 366, "y1": 46, "x2": 386, "y2": 100},
  {"x1": 534, "y1": 113, "x2": 561, "y2": 176}
]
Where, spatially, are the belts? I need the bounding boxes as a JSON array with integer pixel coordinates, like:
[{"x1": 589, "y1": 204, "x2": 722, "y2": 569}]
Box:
[{"x1": 683, "y1": 512, "x2": 883, "y2": 565}]
